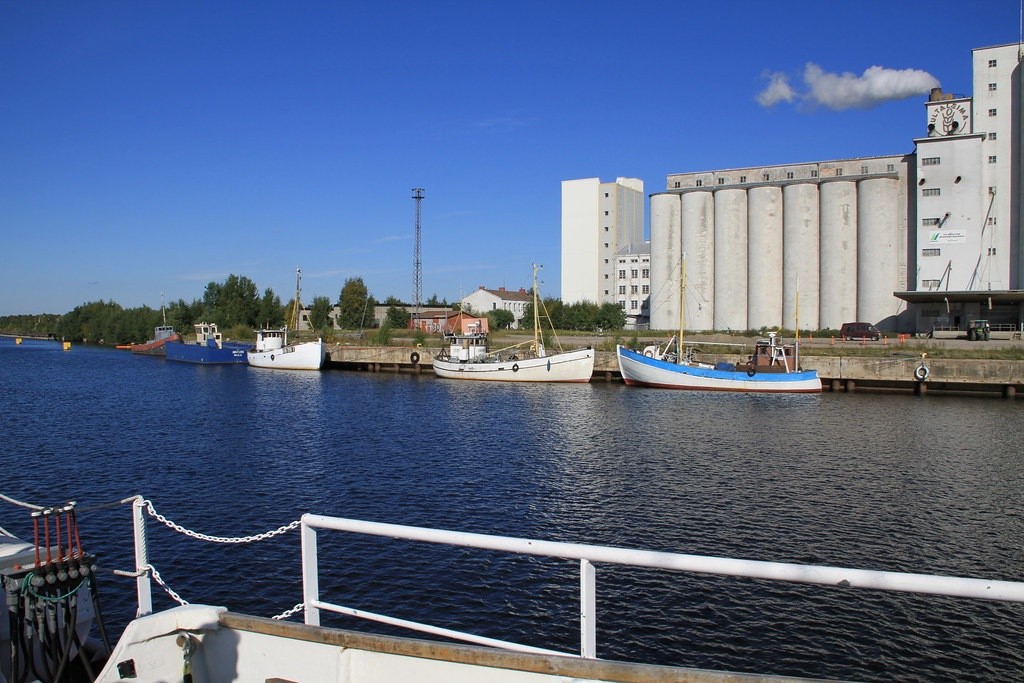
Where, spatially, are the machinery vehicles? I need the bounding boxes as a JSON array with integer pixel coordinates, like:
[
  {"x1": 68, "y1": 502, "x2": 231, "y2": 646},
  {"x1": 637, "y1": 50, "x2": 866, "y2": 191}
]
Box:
[{"x1": 968, "y1": 320, "x2": 990, "y2": 341}]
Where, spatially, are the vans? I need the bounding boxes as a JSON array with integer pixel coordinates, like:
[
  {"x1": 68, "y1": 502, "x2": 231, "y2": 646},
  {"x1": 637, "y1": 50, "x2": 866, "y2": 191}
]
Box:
[{"x1": 840, "y1": 322, "x2": 880, "y2": 341}]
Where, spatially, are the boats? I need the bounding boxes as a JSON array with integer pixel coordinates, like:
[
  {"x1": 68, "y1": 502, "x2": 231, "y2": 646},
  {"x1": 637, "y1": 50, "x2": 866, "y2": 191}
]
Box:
[
  {"x1": 246, "y1": 268, "x2": 328, "y2": 370},
  {"x1": 131, "y1": 293, "x2": 183, "y2": 355},
  {"x1": 0, "y1": 493, "x2": 116, "y2": 683},
  {"x1": 165, "y1": 321, "x2": 251, "y2": 364},
  {"x1": 616, "y1": 254, "x2": 823, "y2": 393},
  {"x1": 432, "y1": 262, "x2": 595, "y2": 383}
]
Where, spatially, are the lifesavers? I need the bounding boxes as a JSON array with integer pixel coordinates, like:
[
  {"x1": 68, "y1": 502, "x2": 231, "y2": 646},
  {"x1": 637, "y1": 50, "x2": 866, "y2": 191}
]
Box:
[
  {"x1": 411, "y1": 352, "x2": 419, "y2": 362},
  {"x1": 513, "y1": 364, "x2": 518, "y2": 371},
  {"x1": 915, "y1": 366, "x2": 929, "y2": 379},
  {"x1": 645, "y1": 350, "x2": 653, "y2": 358},
  {"x1": 271, "y1": 355, "x2": 274, "y2": 359},
  {"x1": 747, "y1": 368, "x2": 756, "y2": 376}
]
